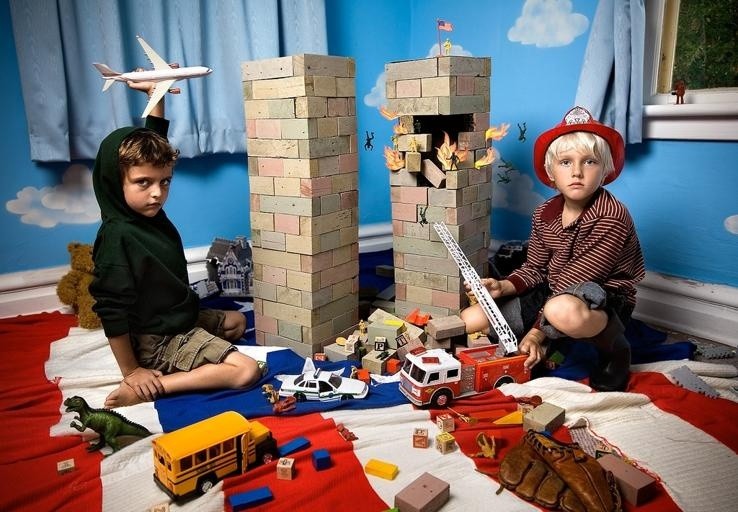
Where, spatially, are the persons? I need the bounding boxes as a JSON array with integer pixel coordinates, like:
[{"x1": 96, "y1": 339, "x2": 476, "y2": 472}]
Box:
[
  {"x1": 87, "y1": 67, "x2": 261, "y2": 410},
  {"x1": 461, "y1": 106, "x2": 646, "y2": 392}
]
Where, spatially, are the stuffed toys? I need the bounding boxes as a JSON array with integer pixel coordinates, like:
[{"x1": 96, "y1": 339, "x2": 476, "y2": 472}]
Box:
[
  {"x1": 57, "y1": 241, "x2": 102, "y2": 330},
  {"x1": 674, "y1": 79, "x2": 685, "y2": 104}
]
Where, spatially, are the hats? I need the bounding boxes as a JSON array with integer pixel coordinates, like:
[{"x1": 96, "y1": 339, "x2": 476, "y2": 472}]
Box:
[{"x1": 534, "y1": 107, "x2": 624, "y2": 189}]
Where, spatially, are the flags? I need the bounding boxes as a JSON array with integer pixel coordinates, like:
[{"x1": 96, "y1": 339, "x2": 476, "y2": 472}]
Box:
[{"x1": 437, "y1": 19, "x2": 453, "y2": 32}]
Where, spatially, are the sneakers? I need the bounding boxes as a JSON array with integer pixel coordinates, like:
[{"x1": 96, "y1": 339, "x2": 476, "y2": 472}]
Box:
[{"x1": 590, "y1": 339, "x2": 631, "y2": 391}]
